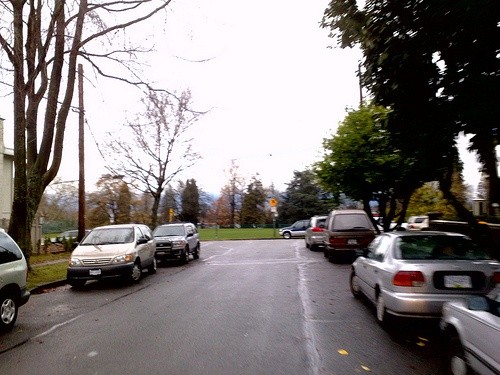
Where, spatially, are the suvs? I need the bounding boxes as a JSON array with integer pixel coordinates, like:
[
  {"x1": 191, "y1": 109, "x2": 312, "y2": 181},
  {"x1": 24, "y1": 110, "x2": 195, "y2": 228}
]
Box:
[
  {"x1": 279, "y1": 220, "x2": 310, "y2": 239},
  {"x1": 152, "y1": 223, "x2": 201, "y2": 264},
  {"x1": 322, "y1": 210, "x2": 376, "y2": 261}
]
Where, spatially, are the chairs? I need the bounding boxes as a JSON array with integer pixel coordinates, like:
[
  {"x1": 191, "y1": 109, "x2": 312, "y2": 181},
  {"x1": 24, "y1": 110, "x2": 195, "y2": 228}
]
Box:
[{"x1": 432, "y1": 242, "x2": 458, "y2": 257}]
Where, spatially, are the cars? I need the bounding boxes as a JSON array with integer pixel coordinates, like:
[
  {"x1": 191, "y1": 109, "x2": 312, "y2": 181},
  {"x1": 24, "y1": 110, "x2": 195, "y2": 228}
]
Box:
[
  {"x1": 407, "y1": 216, "x2": 428, "y2": 231},
  {"x1": 305, "y1": 216, "x2": 329, "y2": 249},
  {"x1": 0, "y1": 228, "x2": 30, "y2": 332},
  {"x1": 66, "y1": 223, "x2": 158, "y2": 288},
  {"x1": 348, "y1": 231, "x2": 500, "y2": 327},
  {"x1": 440, "y1": 282, "x2": 500, "y2": 375}
]
